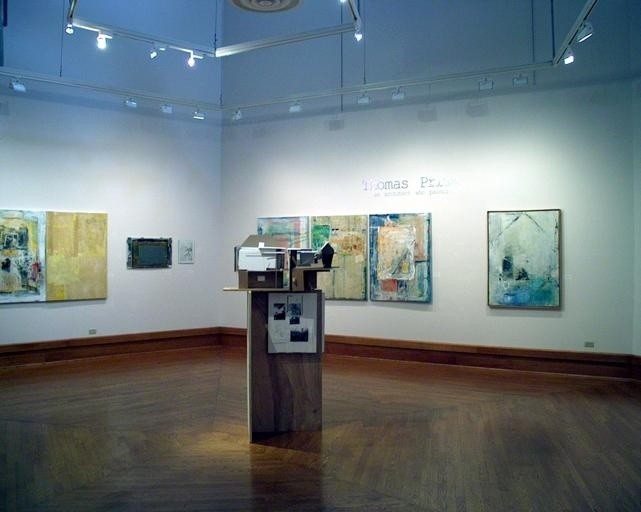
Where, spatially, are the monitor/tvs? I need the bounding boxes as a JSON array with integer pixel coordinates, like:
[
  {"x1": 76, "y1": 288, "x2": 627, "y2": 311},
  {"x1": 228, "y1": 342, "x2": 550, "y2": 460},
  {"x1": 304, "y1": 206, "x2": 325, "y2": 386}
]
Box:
[{"x1": 132, "y1": 238, "x2": 172, "y2": 268}]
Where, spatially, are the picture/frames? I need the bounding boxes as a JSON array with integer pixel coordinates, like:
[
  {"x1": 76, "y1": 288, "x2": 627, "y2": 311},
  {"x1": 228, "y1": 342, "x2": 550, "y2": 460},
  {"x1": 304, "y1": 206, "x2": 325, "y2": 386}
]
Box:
[{"x1": 488, "y1": 209, "x2": 562, "y2": 309}]
